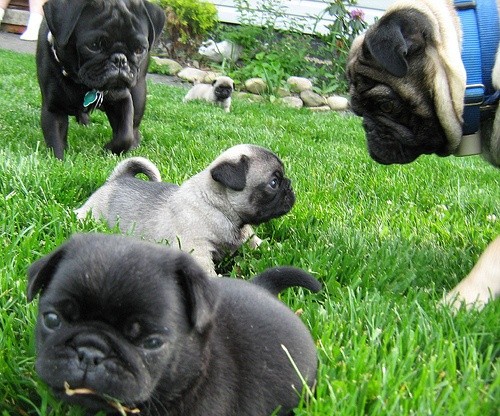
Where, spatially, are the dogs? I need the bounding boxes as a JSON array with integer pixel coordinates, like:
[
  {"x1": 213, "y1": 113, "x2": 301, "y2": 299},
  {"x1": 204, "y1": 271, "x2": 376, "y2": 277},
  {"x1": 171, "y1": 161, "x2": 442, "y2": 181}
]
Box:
[
  {"x1": 182, "y1": 75, "x2": 237, "y2": 114},
  {"x1": 344, "y1": 0, "x2": 500, "y2": 315},
  {"x1": 35, "y1": 0, "x2": 165, "y2": 159},
  {"x1": 71, "y1": 144, "x2": 296, "y2": 279},
  {"x1": 26, "y1": 232, "x2": 323, "y2": 416}
]
